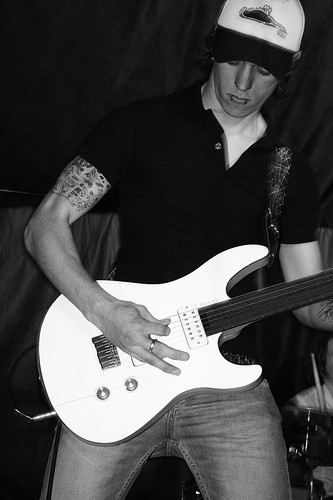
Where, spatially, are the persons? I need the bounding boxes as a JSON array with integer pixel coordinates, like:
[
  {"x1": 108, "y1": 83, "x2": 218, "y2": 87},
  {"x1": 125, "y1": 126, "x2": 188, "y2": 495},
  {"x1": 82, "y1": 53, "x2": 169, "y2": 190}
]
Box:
[
  {"x1": 22, "y1": 1, "x2": 332, "y2": 500},
  {"x1": 283, "y1": 330, "x2": 332, "y2": 500}
]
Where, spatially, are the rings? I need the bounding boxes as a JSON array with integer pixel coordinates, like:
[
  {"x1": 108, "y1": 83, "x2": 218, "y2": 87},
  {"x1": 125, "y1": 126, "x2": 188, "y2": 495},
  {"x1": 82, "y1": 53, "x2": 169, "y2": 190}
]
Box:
[{"x1": 148, "y1": 339, "x2": 157, "y2": 353}]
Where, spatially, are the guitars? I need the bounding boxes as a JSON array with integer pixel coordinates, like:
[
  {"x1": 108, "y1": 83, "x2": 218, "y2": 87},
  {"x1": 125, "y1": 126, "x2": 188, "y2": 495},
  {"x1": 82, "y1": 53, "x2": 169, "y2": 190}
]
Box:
[{"x1": 38, "y1": 246, "x2": 332, "y2": 446}]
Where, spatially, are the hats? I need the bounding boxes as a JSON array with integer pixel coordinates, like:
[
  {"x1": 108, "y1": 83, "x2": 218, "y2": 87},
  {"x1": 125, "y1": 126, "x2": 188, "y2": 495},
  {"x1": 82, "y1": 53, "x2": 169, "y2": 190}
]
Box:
[{"x1": 212, "y1": 0, "x2": 306, "y2": 83}]
